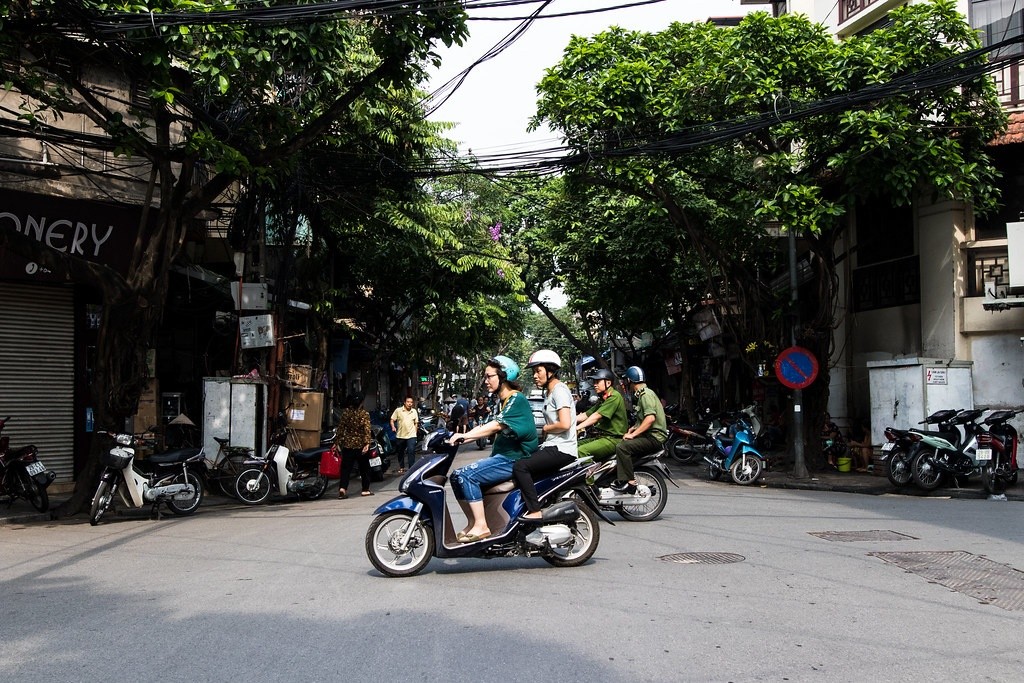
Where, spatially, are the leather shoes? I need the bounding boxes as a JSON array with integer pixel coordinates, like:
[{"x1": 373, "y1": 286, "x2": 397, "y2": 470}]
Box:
[{"x1": 610, "y1": 481, "x2": 637, "y2": 495}]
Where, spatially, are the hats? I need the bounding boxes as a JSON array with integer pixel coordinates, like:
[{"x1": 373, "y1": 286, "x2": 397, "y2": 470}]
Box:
[
  {"x1": 168, "y1": 413, "x2": 195, "y2": 425},
  {"x1": 443, "y1": 396, "x2": 456, "y2": 403}
]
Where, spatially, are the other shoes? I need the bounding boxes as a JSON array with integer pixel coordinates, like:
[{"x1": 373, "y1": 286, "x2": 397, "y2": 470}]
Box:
[
  {"x1": 587, "y1": 485, "x2": 600, "y2": 498},
  {"x1": 457, "y1": 532, "x2": 491, "y2": 542},
  {"x1": 517, "y1": 511, "x2": 542, "y2": 522},
  {"x1": 456, "y1": 532, "x2": 465, "y2": 540}
]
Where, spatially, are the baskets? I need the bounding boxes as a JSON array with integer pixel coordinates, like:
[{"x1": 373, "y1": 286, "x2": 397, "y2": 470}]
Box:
[{"x1": 106, "y1": 448, "x2": 134, "y2": 469}]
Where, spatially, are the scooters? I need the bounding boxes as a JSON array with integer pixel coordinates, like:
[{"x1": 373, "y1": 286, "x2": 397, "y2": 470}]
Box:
[
  {"x1": 471, "y1": 406, "x2": 495, "y2": 449},
  {"x1": 415, "y1": 407, "x2": 447, "y2": 444},
  {"x1": 90, "y1": 427, "x2": 208, "y2": 526},
  {"x1": 234, "y1": 425, "x2": 335, "y2": 507},
  {"x1": 360, "y1": 401, "x2": 619, "y2": 577}
]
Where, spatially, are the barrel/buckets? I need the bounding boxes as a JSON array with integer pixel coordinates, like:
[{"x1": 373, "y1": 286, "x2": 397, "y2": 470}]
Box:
[{"x1": 836, "y1": 458, "x2": 852, "y2": 471}]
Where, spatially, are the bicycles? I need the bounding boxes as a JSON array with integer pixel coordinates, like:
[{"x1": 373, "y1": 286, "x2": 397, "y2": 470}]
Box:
[{"x1": 178, "y1": 427, "x2": 262, "y2": 499}]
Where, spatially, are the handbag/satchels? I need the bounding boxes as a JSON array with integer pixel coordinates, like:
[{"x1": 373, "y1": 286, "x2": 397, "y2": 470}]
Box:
[{"x1": 320, "y1": 448, "x2": 341, "y2": 478}]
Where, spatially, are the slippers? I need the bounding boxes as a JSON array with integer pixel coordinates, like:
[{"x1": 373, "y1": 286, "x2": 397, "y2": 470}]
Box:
[
  {"x1": 338, "y1": 494, "x2": 348, "y2": 498},
  {"x1": 361, "y1": 490, "x2": 374, "y2": 496}
]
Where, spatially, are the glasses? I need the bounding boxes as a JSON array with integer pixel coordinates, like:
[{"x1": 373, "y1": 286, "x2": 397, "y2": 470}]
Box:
[{"x1": 483, "y1": 373, "x2": 498, "y2": 380}]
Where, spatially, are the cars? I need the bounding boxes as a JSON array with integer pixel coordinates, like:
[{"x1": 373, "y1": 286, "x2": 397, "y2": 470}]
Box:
[{"x1": 526, "y1": 389, "x2": 547, "y2": 438}]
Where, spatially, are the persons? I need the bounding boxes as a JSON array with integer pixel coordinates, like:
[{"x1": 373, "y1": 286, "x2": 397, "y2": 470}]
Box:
[
  {"x1": 513, "y1": 348, "x2": 579, "y2": 524},
  {"x1": 609, "y1": 365, "x2": 668, "y2": 496},
  {"x1": 576, "y1": 369, "x2": 627, "y2": 497},
  {"x1": 332, "y1": 391, "x2": 375, "y2": 499},
  {"x1": 389, "y1": 397, "x2": 418, "y2": 474},
  {"x1": 431, "y1": 394, "x2": 494, "y2": 434},
  {"x1": 449, "y1": 355, "x2": 539, "y2": 543}
]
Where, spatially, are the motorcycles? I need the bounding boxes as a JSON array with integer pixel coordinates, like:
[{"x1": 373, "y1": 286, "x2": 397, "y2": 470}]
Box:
[
  {"x1": 659, "y1": 399, "x2": 768, "y2": 487},
  {"x1": 0, "y1": 416, "x2": 57, "y2": 513},
  {"x1": 577, "y1": 430, "x2": 682, "y2": 523},
  {"x1": 363, "y1": 422, "x2": 394, "y2": 483},
  {"x1": 878, "y1": 406, "x2": 1023, "y2": 497}
]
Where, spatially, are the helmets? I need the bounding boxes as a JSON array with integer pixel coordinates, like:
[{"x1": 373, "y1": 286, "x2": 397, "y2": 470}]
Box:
[
  {"x1": 622, "y1": 366, "x2": 643, "y2": 382},
  {"x1": 587, "y1": 369, "x2": 614, "y2": 386},
  {"x1": 493, "y1": 355, "x2": 520, "y2": 382},
  {"x1": 524, "y1": 349, "x2": 561, "y2": 369}
]
